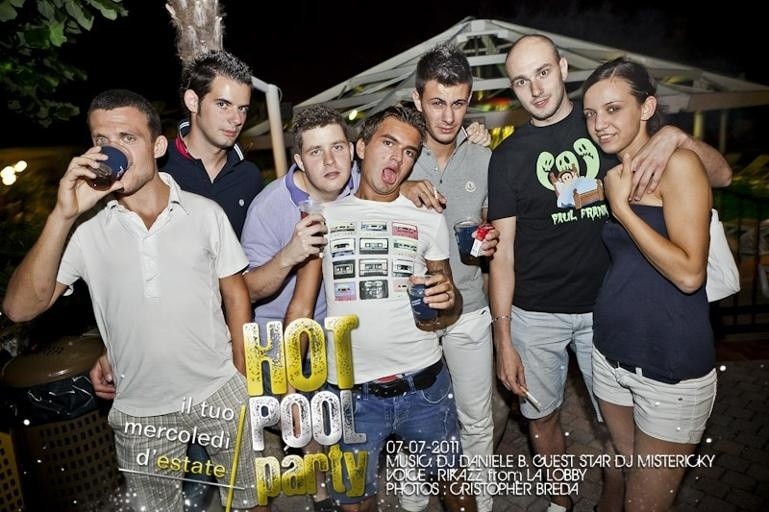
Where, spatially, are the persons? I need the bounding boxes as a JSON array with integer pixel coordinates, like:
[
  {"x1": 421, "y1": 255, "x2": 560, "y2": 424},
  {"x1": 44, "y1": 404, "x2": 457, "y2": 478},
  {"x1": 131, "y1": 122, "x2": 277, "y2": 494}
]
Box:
[
  {"x1": 89, "y1": 48, "x2": 264, "y2": 512},
  {"x1": 1, "y1": 87, "x2": 272, "y2": 512},
  {"x1": 581, "y1": 51, "x2": 718, "y2": 512},
  {"x1": 276, "y1": 104, "x2": 478, "y2": 512},
  {"x1": 485, "y1": 32, "x2": 735, "y2": 512},
  {"x1": 238, "y1": 102, "x2": 493, "y2": 512},
  {"x1": 395, "y1": 42, "x2": 500, "y2": 512}
]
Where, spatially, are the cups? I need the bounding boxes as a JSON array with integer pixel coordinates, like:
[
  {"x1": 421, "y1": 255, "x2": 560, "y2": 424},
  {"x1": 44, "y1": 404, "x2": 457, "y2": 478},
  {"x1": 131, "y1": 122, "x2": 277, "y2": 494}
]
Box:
[
  {"x1": 85, "y1": 142, "x2": 132, "y2": 190},
  {"x1": 454, "y1": 217, "x2": 482, "y2": 265},
  {"x1": 404, "y1": 276, "x2": 440, "y2": 331},
  {"x1": 297, "y1": 201, "x2": 324, "y2": 251}
]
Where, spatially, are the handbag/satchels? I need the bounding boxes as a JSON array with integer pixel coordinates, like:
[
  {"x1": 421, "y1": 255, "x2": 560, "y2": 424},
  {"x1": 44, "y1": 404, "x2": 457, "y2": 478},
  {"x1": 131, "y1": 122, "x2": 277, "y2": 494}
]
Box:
[{"x1": 704, "y1": 209, "x2": 741, "y2": 302}]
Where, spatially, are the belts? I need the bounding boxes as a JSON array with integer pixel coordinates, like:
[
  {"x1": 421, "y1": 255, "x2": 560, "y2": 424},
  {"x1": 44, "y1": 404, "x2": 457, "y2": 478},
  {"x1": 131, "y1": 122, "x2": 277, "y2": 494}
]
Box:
[
  {"x1": 603, "y1": 356, "x2": 680, "y2": 384},
  {"x1": 327, "y1": 358, "x2": 443, "y2": 398}
]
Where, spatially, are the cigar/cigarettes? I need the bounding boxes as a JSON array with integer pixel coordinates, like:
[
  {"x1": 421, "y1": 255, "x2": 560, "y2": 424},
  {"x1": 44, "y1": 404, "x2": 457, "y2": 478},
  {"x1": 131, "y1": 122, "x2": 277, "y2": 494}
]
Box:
[
  {"x1": 432, "y1": 185, "x2": 441, "y2": 201},
  {"x1": 519, "y1": 385, "x2": 545, "y2": 410}
]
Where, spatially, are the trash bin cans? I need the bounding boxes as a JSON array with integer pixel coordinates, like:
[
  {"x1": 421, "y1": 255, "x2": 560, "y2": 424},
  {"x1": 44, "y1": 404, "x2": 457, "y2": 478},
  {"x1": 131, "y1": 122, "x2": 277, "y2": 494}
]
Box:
[{"x1": 0, "y1": 335, "x2": 125, "y2": 512}]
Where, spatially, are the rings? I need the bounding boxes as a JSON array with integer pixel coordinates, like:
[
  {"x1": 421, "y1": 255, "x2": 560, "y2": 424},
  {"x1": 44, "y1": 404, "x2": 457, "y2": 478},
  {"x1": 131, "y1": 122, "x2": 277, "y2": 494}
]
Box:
[{"x1": 445, "y1": 291, "x2": 453, "y2": 302}]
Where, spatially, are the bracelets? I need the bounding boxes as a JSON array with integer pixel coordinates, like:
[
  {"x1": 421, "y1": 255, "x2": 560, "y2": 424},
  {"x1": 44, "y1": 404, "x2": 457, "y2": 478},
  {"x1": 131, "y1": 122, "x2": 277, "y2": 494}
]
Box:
[{"x1": 490, "y1": 312, "x2": 514, "y2": 326}]
